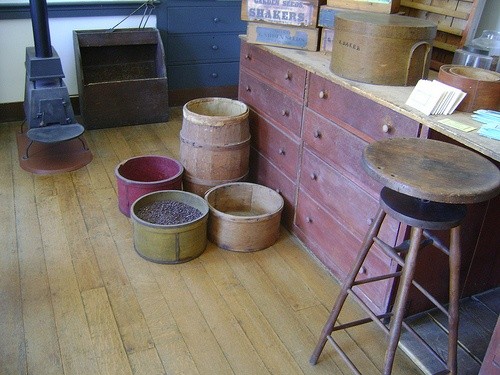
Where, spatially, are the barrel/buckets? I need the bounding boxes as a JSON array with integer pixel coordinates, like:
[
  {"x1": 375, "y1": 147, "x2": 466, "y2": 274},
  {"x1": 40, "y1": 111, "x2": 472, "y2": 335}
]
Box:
[{"x1": 178, "y1": 96, "x2": 252, "y2": 199}]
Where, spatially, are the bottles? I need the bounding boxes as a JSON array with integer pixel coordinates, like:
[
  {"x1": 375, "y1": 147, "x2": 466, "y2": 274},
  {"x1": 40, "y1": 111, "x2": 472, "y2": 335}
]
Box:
[
  {"x1": 452, "y1": 43, "x2": 493, "y2": 70},
  {"x1": 471, "y1": 29, "x2": 500, "y2": 73}
]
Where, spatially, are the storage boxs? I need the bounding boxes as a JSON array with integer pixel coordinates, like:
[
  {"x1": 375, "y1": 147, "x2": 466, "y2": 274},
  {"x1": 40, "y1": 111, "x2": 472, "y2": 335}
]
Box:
[
  {"x1": 241, "y1": 0, "x2": 405, "y2": 53},
  {"x1": 73, "y1": 28, "x2": 170, "y2": 131}
]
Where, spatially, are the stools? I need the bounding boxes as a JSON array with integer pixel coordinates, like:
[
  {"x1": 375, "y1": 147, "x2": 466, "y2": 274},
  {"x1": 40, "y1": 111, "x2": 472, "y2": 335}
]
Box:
[{"x1": 307, "y1": 136, "x2": 500, "y2": 375}]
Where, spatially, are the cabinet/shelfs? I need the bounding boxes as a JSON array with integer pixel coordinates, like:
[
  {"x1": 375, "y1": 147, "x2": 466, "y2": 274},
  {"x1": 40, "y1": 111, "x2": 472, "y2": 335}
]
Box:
[{"x1": 237, "y1": 33, "x2": 500, "y2": 324}]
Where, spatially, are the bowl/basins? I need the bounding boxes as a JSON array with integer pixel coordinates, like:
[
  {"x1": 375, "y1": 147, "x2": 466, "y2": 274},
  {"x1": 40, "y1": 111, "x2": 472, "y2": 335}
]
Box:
[
  {"x1": 204, "y1": 182, "x2": 285, "y2": 253},
  {"x1": 129, "y1": 190, "x2": 210, "y2": 265},
  {"x1": 329, "y1": 11, "x2": 439, "y2": 86},
  {"x1": 114, "y1": 155, "x2": 183, "y2": 218}
]
